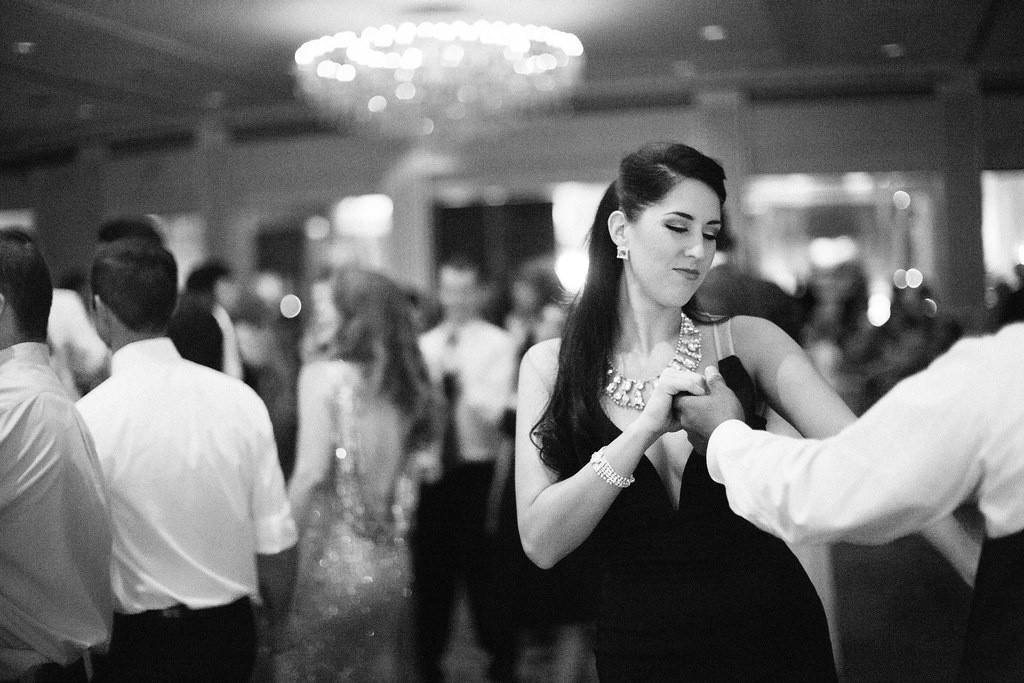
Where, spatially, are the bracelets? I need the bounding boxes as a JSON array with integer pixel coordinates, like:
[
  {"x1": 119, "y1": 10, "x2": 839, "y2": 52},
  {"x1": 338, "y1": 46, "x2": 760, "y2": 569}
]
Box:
[{"x1": 588, "y1": 447, "x2": 635, "y2": 488}]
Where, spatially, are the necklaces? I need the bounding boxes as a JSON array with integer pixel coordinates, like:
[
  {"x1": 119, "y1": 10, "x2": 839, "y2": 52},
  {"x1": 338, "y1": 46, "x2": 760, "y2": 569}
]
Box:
[{"x1": 604, "y1": 312, "x2": 701, "y2": 410}]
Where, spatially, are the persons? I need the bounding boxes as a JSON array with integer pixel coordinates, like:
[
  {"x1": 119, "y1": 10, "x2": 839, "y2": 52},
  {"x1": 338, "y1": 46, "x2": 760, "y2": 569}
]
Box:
[
  {"x1": 0, "y1": 215, "x2": 1022, "y2": 682},
  {"x1": 673, "y1": 319, "x2": 1024, "y2": 683},
  {"x1": 516, "y1": 141, "x2": 982, "y2": 683}
]
